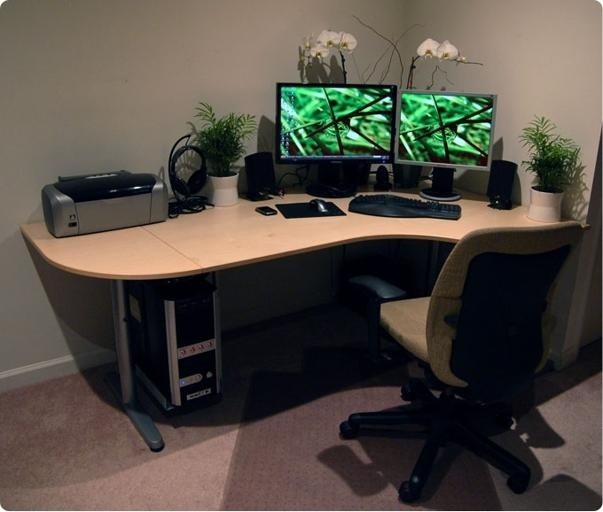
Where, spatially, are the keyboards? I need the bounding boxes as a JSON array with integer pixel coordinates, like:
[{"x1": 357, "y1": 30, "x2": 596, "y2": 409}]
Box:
[{"x1": 347, "y1": 195, "x2": 461, "y2": 220}]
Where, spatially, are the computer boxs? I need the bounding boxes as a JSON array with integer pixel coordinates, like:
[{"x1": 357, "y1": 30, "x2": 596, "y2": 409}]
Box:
[
  {"x1": 331, "y1": 268, "x2": 408, "y2": 373},
  {"x1": 135, "y1": 279, "x2": 223, "y2": 408}
]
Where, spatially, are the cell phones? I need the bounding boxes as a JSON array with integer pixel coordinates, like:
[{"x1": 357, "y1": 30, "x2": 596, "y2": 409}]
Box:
[{"x1": 255, "y1": 206, "x2": 278, "y2": 216}]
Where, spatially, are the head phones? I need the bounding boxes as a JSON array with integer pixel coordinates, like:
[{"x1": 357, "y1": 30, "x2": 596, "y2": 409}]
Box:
[{"x1": 169, "y1": 144, "x2": 209, "y2": 196}]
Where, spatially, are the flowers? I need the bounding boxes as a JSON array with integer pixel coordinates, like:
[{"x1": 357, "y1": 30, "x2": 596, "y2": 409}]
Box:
[{"x1": 301, "y1": 15, "x2": 483, "y2": 91}]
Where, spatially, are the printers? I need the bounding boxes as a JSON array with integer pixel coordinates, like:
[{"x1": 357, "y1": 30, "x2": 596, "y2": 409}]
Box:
[{"x1": 41, "y1": 170, "x2": 170, "y2": 238}]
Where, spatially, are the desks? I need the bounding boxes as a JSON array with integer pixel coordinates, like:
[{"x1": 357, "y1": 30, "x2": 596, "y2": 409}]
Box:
[{"x1": 19, "y1": 170, "x2": 592, "y2": 452}]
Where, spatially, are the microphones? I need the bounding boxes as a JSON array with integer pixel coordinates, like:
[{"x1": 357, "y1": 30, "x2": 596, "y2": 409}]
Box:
[{"x1": 176, "y1": 135, "x2": 191, "y2": 138}]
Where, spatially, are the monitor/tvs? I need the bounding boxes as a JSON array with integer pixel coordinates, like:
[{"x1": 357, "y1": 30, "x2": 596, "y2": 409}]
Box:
[
  {"x1": 275, "y1": 81, "x2": 398, "y2": 198},
  {"x1": 394, "y1": 89, "x2": 498, "y2": 202}
]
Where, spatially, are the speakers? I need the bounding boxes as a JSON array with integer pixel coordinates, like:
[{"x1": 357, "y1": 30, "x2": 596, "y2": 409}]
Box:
[
  {"x1": 486, "y1": 160, "x2": 518, "y2": 210},
  {"x1": 244, "y1": 151, "x2": 276, "y2": 202}
]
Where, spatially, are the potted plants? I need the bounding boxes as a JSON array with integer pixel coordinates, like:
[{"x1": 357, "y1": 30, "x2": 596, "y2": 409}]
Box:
[
  {"x1": 518, "y1": 113, "x2": 582, "y2": 223},
  {"x1": 191, "y1": 100, "x2": 259, "y2": 207}
]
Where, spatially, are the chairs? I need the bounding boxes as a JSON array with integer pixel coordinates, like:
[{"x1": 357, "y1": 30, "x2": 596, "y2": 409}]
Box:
[{"x1": 340, "y1": 220, "x2": 583, "y2": 503}]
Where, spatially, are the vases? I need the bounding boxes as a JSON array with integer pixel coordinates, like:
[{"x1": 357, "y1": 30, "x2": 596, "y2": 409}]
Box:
[
  {"x1": 343, "y1": 163, "x2": 371, "y2": 186},
  {"x1": 392, "y1": 163, "x2": 422, "y2": 188}
]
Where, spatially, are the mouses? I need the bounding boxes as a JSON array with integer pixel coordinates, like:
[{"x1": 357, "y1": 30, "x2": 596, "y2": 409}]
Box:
[{"x1": 309, "y1": 199, "x2": 329, "y2": 213}]
